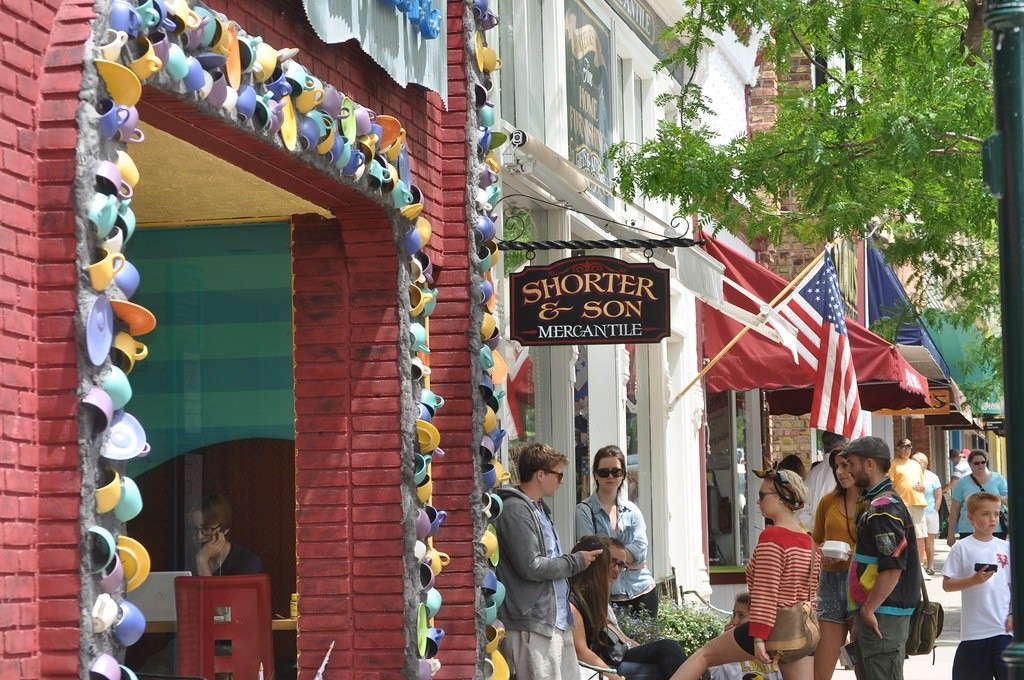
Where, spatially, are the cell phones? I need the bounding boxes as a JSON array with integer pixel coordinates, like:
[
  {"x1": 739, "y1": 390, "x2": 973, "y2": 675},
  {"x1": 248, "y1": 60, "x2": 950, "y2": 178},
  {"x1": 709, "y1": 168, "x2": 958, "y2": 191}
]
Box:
[{"x1": 974, "y1": 563, "x2": 997, "y2": 572}]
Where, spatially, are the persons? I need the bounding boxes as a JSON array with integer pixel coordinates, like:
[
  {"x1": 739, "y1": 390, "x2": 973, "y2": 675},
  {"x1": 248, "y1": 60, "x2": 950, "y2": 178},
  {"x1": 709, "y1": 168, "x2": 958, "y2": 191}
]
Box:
[
  {"x1": 495, "y1": 444, "x2": 604, "y2": 680},
  {"x1": 567, "y1": 535, "x2": 700, "y2": 680},
  {"x1": 838, "y1": 436, "x2": 922, "y2": 680},
  {"x1": 812, "y1": 442, "x2": 859, "y2": 680},
  {"x1": 669, "y1": 469, "x2": 821, "y2": 680},
  {"x1": 577, "y1": 446, "x2": 659, "y2": 619},
  {"x1": 163, "y1": 493, "x2": 261, "y2": 576},
  {"x1": 942, "y1": 448, "x2": 1008, "y2": 547},
  {"x1": 777, "y1": 432, "x2": 846, "y2": 537},
  {"x1": 886, "y1": 438, "x2": 942, "y2": 581},
  {"x1": 725, "y1": 593, "x2": 751, "y2": 631},
  {"x1": 940, "y1": 492, "x2": 1014, "y2": 680}
]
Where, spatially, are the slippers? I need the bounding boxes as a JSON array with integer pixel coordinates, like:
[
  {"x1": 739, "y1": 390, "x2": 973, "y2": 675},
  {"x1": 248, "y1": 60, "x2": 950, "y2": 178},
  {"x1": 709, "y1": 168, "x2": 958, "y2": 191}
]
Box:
[{"x1": 925, "y1": 568, "x2": 934, "y2": 575}]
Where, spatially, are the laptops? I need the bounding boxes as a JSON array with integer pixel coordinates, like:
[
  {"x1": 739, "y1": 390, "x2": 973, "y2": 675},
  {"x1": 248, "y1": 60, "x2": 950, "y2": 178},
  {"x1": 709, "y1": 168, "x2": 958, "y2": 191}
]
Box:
[{"x1": 124, "y1": 570, "x2": 191, "y2": 622}]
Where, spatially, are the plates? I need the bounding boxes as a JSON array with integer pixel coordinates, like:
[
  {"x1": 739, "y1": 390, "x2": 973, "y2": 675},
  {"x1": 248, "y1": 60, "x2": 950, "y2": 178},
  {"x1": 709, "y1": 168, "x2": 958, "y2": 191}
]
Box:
[
  {"x1": 107, "y1": 300, "x2": 158, "y2": 336},
  {"x1": 482, "y1": 270, "x2": 496, "y2": 313},
  {"x1": 490, "y1": 349, "x2": 508, "y2": 383},
  {"x1": 92, "y1": 55, "x2": 142, "y2": 107},
  {"x1": 473, "y1": 29, "x2": 486, "y2": 72},
  {"x1": 416, "y1": 602, "x2": 428, "y2": 659},
  {"x1": 369, "y1": 113, "x2": 402, "y2": 151},
  {"x1": 100, "y1": 411, "x2": 148, "y2": 459},
  {"x1": 413, "y1": 418, "x2": 440, "y2": 453},
  {"x1": 487, "y1": 521, "x2": 499, "y2": 566},
  {"x1": 487, "y1": 131, "x2": 507, "y2": 150},
  {"x1": 402, "y1": 203, "x2": 423, "y2": 219},
  {"x1": 85, "y1": 294, "x2": 114, "y2": 366},
  {"x1": 489, "y1": 648, "x2": 511, "y2": 680},
  {"x1": 278, "y1": 91, "x2": 299, "y2": 151},
  {"x1": 224, "y1": 22, "x2": 242, "y2": 90},
  {"x1": 340, "y1": 96, "x2": 357, "y2": 145},
  {"x1": 115, "y1": 534, "x2": 151, "y2": 593}
]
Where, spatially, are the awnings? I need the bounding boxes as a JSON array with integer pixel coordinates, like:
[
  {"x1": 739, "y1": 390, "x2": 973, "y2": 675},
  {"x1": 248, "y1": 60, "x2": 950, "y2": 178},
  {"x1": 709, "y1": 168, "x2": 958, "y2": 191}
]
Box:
[
  {"x1": 667, "y1": 224, "x2": 1005, "y2": 431},
  {"x1": 510, "y1": 130, "x2": 727, "y2": 308}
]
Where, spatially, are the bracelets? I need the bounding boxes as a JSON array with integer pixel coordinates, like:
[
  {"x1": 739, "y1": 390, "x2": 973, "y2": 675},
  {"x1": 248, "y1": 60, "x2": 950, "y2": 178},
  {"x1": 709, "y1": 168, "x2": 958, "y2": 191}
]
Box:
[{"x1": 753, "y1": 639, "x2": 764, "y2": 643}]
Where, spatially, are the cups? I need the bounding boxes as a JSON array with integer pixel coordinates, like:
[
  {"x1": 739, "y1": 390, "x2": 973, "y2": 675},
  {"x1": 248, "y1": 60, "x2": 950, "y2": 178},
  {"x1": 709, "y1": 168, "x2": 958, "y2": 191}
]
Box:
[{"x1": 77, "y1": 0, "x2": 514, "y2": 680}]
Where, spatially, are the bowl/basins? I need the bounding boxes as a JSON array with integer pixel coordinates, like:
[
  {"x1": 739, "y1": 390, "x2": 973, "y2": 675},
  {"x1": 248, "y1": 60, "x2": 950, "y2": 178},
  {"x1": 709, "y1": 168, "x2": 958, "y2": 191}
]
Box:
[{"x1": 821, "y1": 540, "x2": 851, "y2": 561}]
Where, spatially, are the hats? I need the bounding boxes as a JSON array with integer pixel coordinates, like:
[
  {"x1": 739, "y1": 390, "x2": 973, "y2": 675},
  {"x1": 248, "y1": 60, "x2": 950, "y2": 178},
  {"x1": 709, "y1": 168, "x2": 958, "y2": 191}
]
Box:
[
  {"x1": 960, "y1": 449, "x2": 970, "y2": 455},
  {"x1": 838, "y1": 436, "x2": 891, "y2": 460},
  {"x1": 948, "y1": 448, "x2": 961, "y2": 459},
  {"x1": 897, "y1": 438, "x2": 912, "y2": 447}
]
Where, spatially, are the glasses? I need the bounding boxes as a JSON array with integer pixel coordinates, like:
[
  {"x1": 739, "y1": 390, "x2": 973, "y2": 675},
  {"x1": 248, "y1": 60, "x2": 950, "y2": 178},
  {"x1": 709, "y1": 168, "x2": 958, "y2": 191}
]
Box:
[
  {"x1": 758, "y1": 491, "x2": 778, "y2": 502},
  {"x1": 534, "y1": 467, "x2": 564, "y2": 483},
  {"x1": 595, "y1": 469, "x2": 625, "y2": 478},
  {"x1": 970, "y1": 461, "x2": 986, "y2": 465},
  {"x1": 899, "y1": 445, "x2": 912, "y2": 450},
  {"x1": 186, "y1": 524, "x2": 220, "y2": 537},
  {"x1": 610, "y1": 559, "x2": 628, "y2": 572}
]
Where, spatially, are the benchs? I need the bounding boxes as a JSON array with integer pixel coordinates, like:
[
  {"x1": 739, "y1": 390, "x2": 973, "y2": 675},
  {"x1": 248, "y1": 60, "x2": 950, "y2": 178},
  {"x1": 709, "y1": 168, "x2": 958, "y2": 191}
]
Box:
[{"x1": 579, "y1": 566, "x2": 734, "y2": 680}]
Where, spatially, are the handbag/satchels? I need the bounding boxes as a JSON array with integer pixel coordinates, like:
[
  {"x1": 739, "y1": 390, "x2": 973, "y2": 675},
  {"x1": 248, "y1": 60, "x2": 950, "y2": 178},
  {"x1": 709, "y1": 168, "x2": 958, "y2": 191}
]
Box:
[
  {"x1": 904, "y1": 601, "x2": 945, "y2": 665},
  {"x1": 1000, "y1": 511, "x2": 1009, "y2": 535},
  {"x1": 763, "y1": 599, "x2": 822, "y2": 672},
  {"x1": 599, "y1": 627, "x2": 628, "y2": 667}
]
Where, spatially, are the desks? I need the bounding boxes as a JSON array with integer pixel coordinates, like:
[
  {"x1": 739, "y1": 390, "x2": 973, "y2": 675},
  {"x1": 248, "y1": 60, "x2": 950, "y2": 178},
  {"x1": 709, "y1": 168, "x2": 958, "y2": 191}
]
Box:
[{"x1": 122, "y1": 619, "x2": 298, "y2": 680}]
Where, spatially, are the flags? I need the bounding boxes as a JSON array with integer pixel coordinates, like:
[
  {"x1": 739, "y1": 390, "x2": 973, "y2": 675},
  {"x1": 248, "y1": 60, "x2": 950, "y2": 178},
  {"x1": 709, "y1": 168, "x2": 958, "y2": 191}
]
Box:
[{"x1": 764, "y1": 246, "x2": 865, "y2": 443}]
